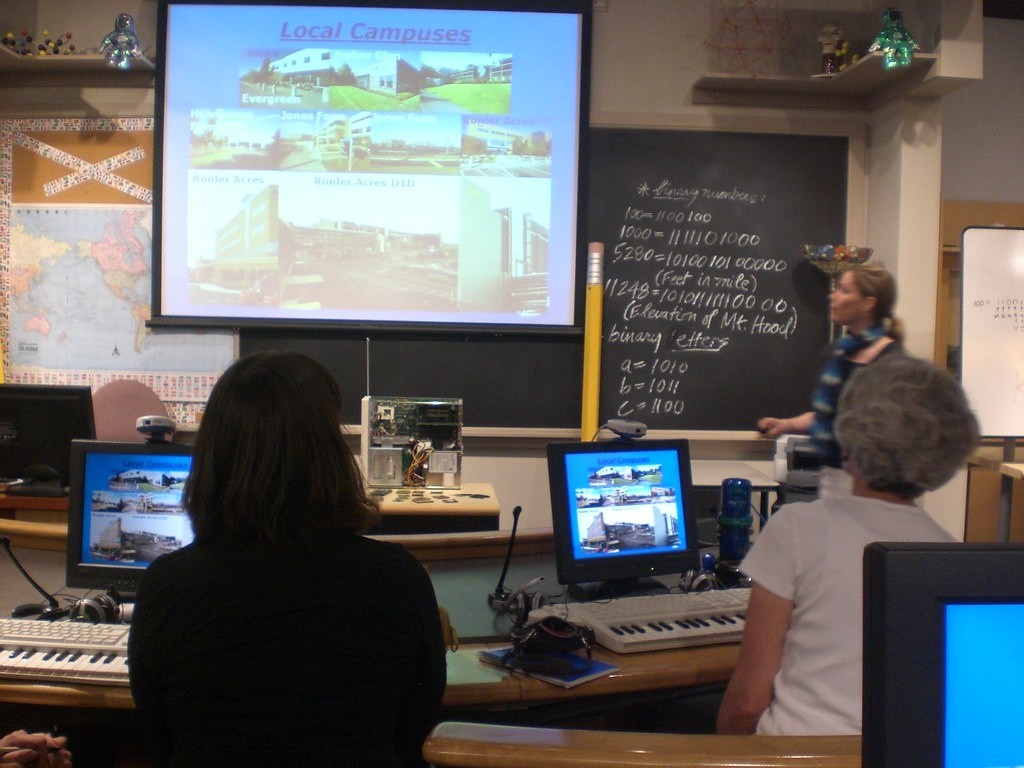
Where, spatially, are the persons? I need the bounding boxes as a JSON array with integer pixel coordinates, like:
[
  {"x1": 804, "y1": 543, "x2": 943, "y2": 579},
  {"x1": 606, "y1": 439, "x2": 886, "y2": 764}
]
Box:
[
  {"x1": 128, "y1": 354, "x2": 448, "y2": 768},
  {"x1": 868, "y1": 6, "x2": 920, "y2": 69},
  {"x1": 0, "y1": 730, "x2": 72, "y2": 768},
  {"x1": 757, "y1": 264, "x2": 907, "y2": 497},
  {"x1": 718, "y1": 354, "x2": 981, "y2": 735},
  {"x1": 99, "y1": 13, "x2": 141, "y2": 69}
]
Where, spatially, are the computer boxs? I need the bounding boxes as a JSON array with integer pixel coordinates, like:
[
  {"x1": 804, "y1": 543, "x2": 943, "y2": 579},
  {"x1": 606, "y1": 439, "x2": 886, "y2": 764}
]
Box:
[{"x1": 361, "y1": 395, "x2": 463, "y2": 490}]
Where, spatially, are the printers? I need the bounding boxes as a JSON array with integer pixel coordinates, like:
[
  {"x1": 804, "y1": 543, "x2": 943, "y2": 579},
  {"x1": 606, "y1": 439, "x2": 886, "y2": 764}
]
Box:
[{"x1": 772, "y1": 434, "x2": 821, "y2": 487}]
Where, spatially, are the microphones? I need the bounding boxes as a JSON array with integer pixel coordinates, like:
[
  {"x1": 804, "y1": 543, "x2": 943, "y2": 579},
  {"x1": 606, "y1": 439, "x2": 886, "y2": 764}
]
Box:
[
  {"x1": 0, "y1": 537, "x2": 69, "y2": 620},
  {"x1": 488, "y1": 506, "x2": 523, "y2": 606}
]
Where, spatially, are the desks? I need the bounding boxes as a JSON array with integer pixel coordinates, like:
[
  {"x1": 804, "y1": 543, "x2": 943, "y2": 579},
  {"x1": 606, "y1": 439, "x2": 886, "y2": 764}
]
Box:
[
  {"x1": 0, "y1": 480, "x2": 500, "y2": 535},
  {"x1": 0, "y1": 641, "x2": 742, "y2": 708}
]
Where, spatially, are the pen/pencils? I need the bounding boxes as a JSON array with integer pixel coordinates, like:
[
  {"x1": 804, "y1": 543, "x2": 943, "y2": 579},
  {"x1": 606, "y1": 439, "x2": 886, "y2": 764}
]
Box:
[{"x1": 581, "y1": 241, "x2": 603, "y2": 442}]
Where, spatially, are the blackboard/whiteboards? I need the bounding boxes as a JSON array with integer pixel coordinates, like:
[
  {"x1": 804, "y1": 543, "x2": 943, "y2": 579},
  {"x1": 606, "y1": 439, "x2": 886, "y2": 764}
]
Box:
[{"x1": 237, "y1": 110, "x2": 872, "y2": 439}]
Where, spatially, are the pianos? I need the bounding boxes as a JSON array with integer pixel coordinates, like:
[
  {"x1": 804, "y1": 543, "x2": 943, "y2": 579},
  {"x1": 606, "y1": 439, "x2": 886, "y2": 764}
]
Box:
[
  {"x1": 0, "y1": 618, "x2": 131, "y2": 687},
  {"x1": 541, "y1": 587, "x2": 753, "y2": 654}
]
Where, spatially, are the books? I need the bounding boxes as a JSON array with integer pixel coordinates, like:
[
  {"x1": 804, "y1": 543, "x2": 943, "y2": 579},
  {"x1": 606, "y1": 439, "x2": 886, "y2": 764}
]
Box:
[{"x1": 479, "y1": 640, "x2": 621, "y2": 689}]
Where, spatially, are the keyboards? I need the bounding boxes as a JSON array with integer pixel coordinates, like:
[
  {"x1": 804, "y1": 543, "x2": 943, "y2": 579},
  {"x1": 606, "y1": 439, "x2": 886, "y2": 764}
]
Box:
[
  {"x1": 0, "y1": 619, "x2": 131, "y2": 656},
  {"x1": 528, "y1": 588, "x2": 751, "y2": 623}
]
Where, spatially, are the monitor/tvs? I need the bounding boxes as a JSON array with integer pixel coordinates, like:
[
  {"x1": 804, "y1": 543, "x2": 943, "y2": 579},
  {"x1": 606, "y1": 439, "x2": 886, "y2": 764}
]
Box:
[
  {"x1": 862, "y1": 541, "x2": 1024, "y2": 768},
  {"x1": 66, "y1": 439, "x2": 195, "y2": 589},
  {"x1": 547, "y1": 438, "x2": 701, "y2": 601},
  {"x1": 0, "y1": 384, "x2": 96, "y2": 497}
]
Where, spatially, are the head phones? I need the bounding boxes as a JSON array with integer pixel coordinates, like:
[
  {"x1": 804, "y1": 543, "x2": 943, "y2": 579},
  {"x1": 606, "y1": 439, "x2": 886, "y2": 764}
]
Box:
[
  {"x1": 68, "y1": 594, "x2": 121, "y2": 624},
  {"x1": 678, "y1": 569, "x2": 721, "y2": 591},
  {"x1": 501, "y1": 576, "x2": 549, "y2": 626}
]
